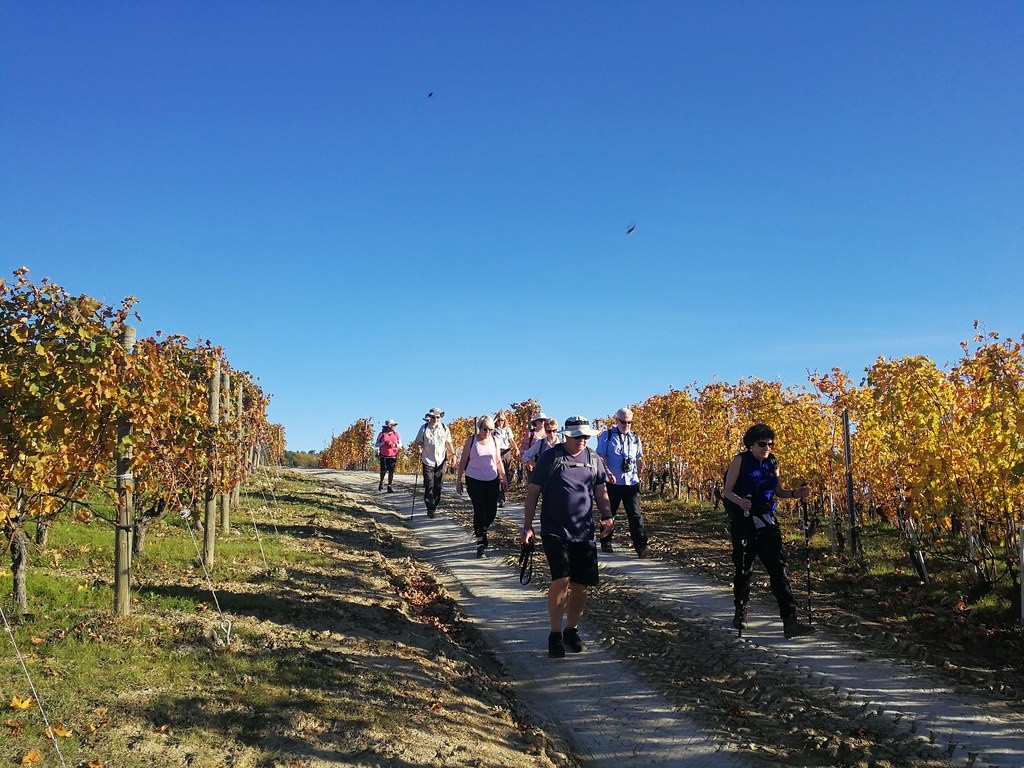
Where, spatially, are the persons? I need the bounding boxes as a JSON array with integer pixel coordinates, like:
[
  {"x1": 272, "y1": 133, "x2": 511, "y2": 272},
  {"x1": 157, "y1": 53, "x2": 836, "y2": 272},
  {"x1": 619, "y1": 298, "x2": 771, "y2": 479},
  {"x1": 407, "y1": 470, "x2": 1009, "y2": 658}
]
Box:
[
  {"x1": 522, "y1": 418, "x2": 561, "y2": 467},
  {"x1": 520, "y1": 416, "x2": 615, "y2": 658},
  {"x1": 520, "y1": 413, "x2": 550, "y2": 486},
  {"x1": 596, "y1": 409, "x2": 651, "y2": 558},
  {"x1": 375, "y1": 419, "x2": 402, "y2": 493},
  {"x1": 414, "y1": 407, "x2": 457, "y2": 519},
  {"x1": 456, "y1": 415, "x2": 509, "y2": 559},
  {"x1": 491, "y1": 410, "x2": 520, "y2": 507},
  {"x1": 723, "y1": 423, "x2": 817, "y2": 639}
]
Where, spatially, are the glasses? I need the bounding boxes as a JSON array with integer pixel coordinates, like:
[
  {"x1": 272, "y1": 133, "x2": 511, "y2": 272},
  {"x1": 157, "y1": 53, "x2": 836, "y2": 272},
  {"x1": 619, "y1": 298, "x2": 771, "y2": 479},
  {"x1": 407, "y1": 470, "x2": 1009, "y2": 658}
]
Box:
[
  {"x1": 573, "y1": 435, "x2": 591, "y2": 439},
  {"x1": 545, "y1": 429, "x2": 557, "y2": 434},
  {"x1": 481, "y1": 428, "x2": 493, "y2": 432},
  {"x1": 431, "y1": 416, "x2": 440, "y2": 419},
  {"x1": 616, "y1": 418, "x2": 632, "y2": 424},
  {"x1": 756, "y1": 441, "x2": 775, "y2": 448}
]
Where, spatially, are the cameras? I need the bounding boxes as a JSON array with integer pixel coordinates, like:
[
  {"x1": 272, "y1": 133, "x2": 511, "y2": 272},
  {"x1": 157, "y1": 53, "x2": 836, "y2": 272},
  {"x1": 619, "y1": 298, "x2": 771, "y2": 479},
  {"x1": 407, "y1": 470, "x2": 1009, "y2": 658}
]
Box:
[{"x1": 621, "y1": 459, "x2": 632, "y2": 473}]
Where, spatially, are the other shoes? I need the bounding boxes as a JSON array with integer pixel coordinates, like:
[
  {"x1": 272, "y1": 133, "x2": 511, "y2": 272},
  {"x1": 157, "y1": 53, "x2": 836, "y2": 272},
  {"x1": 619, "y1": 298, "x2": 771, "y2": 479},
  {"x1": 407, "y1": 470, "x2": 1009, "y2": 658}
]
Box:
[
  {"x1": 638, "y1": 544, "x2": 650, "y2": 559},
  {"x1": 499, "y1": 501, "x2": 505, "y2": 508},
  {"x1": 476, "y1": 545, "x2": 486, "y2": 558},
  {"x1": 379, "y1": 484, "x2": 383, "y2": 491},
  {"x1": 484, "y1": 532, "x2": 489, "y2": 547},
  {"x1": 387, "y1": 486, "x2": 393, "y2": 493},
  {"x1": 601, "y1": 543, "x2": 613, "y2": 552},
  {"x1": 427, "y1": 511, "x2": 435, "y2": 518}
]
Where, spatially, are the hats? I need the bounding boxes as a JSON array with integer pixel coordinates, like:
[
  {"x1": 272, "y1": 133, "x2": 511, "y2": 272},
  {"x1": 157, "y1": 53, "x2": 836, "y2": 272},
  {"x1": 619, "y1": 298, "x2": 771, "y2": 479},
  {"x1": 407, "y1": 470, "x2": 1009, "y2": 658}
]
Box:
[
  {"x1": 382, "y1": 419, "x2": 398, "y2": 427},
  {"x1": 425, "y1": 408, "x2": 444, "y2": 418},
  {"x1": 531, "y1": 413, "x2": 549, "y2": 427},
  {"x1": 422, "y1": 413, "x2": 431, "y2": 420},
  {"x1": 560, "y1": 416, "x2": 601, "y2": 437}
]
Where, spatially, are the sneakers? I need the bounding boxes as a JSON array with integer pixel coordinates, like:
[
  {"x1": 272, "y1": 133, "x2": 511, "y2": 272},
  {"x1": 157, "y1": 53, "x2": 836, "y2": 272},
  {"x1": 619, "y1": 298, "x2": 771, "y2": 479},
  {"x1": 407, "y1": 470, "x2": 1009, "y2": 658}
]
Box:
[
  {"x1": 548, "y1": 635, "x2": 565, "y2": 658},
  {"x1": 733, "y1": 605, "x2": 753, "y2": 629},
  {"x1": 563, "y1": 628, "x2": 587, "y2": 652},
  {"x1": 784, "y1": 615, "x2": 817, "y2": 638}
]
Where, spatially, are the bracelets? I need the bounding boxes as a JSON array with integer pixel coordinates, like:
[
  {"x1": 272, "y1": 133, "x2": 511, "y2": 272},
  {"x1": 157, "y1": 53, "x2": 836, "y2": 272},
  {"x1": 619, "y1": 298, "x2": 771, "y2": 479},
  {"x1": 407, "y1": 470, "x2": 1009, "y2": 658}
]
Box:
[
  {"x1": 601, "y1": 514, "x2": 613, "y2": 520},
  {"x1": 385, "y1": 442, "x2": 386, "y2": 444},
  {"x1": 791, "y1": 490, "x2": 795, "y2": 498},
  {"x1": 452, "y1": 454, "x2": 455, "y2": 455},
  {"x1": 395, "y1": 443, "x2": 397, "y2": 447}
]
